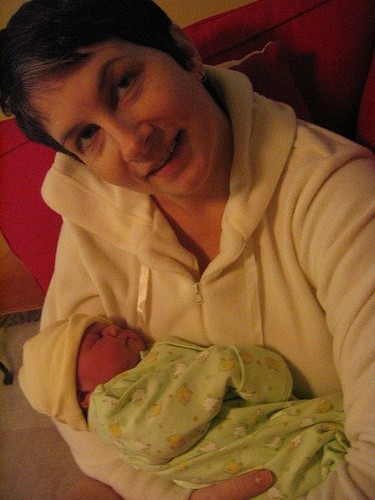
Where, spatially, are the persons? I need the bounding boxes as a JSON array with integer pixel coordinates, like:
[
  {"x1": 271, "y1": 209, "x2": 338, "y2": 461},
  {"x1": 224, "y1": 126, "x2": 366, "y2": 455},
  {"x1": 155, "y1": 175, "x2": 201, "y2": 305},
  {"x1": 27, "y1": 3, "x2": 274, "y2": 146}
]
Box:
[
  {"x1": 16, "y1": 313, "x2": 350, "y2": 499},
  {"x1": 0, "y1": 0, "x2": 375, "y2": 500}
]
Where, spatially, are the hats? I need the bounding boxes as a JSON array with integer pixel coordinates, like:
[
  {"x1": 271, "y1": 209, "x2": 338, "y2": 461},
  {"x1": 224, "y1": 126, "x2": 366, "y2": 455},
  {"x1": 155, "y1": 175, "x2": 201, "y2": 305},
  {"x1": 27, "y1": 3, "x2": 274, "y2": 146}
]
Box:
[{"x1": 16, "y1": 313, "x2": 111, "y2": 432}]
